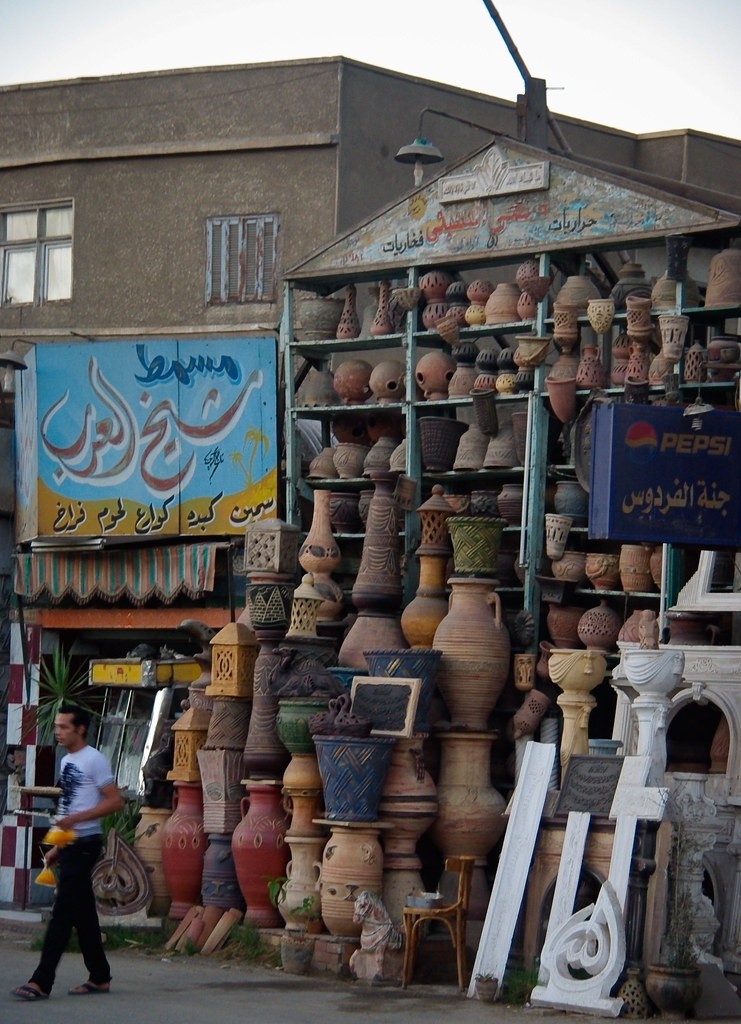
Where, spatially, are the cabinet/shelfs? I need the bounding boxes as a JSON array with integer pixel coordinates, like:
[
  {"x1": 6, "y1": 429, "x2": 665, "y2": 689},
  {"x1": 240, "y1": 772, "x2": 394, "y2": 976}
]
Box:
[{"x1": 270, "y1": 219, "x2": 740, "y2": 691}]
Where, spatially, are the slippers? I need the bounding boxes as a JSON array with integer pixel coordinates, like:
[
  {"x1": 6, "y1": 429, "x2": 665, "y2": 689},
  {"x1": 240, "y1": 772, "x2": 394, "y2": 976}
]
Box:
[
  {"x1": 11, "y1": 986, "x2": 49, "y2": 1000},
  {"x1": 69, "y1": 984, "x2": 110, "y2": 996}
]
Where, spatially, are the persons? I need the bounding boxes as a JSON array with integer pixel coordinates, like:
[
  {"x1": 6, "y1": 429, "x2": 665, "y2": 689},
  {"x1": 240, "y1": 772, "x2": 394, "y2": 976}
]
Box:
[{"x1": 6, "y1": 705, "x2": 128, "y2": 1003}]
